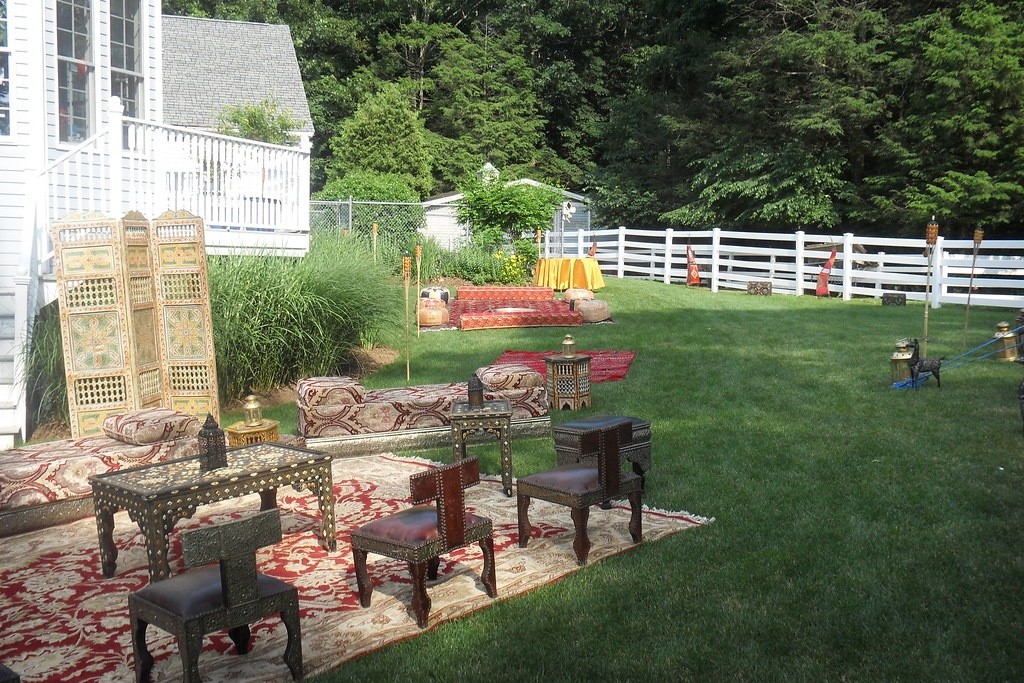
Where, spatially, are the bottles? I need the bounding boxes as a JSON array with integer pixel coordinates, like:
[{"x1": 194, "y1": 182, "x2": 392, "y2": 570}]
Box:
[
  {"x1": 562, "y1": 333, "x2": 576, "y2": 359},
  {"x1": 243, "y1": 395, "x2": 263, "y2": 427}
]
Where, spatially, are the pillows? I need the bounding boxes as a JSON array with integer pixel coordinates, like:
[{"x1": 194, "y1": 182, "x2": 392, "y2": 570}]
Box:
[
  {"x1": 297, "y1": 373, "x2": 368, "y2": 404},
  {"x1": 103, "y1": 404, "x2": 200, "y2": 444},
  {"x1": 475, "y1": 364, "x2": 541, "y2": 389}
]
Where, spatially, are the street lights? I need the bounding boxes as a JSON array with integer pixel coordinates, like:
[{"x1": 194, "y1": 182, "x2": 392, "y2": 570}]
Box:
[
  {"x1": 536, "y1": 229, "x2": 542, "y2": 259},
  {"x1": 402, "y1": 250, "x2": 411, "y2": 383},
  {"x1": 414, "y1": 242, "x2": 421, "y2": 337},
  {"x1": 371, "y1": 221, "x2": 379, "y2": 266}
]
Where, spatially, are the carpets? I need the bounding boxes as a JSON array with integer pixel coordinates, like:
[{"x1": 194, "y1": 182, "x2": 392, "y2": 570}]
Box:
[
  {"x1": 493, "y1": 349, "x2": 638, "y2": 384},
  {"x1": 1, "y1": 447, "x2": 717, "y2": 681}
]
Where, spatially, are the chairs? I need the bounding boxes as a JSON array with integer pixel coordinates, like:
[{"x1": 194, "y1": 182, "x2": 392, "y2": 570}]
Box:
[
  {"x1": 128, "y1": 507, "x2": 304, "y2": 683},
  {"x1": 352, "y1": 456, "x2": 497, "y2": 629},
  {"x1": 516, "y1": 419, "x2": 646, "y2": 567}
]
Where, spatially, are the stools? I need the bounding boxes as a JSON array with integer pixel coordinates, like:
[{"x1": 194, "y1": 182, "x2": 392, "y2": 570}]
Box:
[
  {"x1": 449, "y1": 398, "x2": 514, "y2": 495},
  {"x1": 553, "y1": 415, "x2": 652, "y2": 475}
]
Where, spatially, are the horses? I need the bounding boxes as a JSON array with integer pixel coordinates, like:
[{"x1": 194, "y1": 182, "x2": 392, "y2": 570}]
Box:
[{"x1": 905, "y1": 338, "x2": 945, "y2": 391}]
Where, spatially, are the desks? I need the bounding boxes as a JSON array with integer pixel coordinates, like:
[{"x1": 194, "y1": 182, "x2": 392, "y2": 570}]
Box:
[
  {"x1": 533, "y1": 258, "x2": 604, "y2": 294},
  {"x1": 225, "y1": 418, "x2": 282, "y2": 447},
  {"x1": 545, "y1": 352, "x2": 594, "y2": 409},
  {"x1": 88, "y1": 441, "x2": 336, "y2": 584}
]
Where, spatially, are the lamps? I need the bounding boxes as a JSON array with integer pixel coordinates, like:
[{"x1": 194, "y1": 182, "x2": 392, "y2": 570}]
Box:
[
  {"x1": 561, "y1": 334, "x2": 577, "y2": 359},
  {"x1": 887, "y1": 339, "x2": 913, "y2": 382},
  {"x1": 243, "y1": 395, "x2": 264, "y2": 426},
  {"x1": 989, "y1": 321, "x2": 1021, "y2": 364}
]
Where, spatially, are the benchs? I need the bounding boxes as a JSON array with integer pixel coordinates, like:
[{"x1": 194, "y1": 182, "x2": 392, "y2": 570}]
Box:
[
  {"x1": 456, "y1": 286, "x2": 554, "y2": 299},
  {"x1": 459, "y1": 311, "x2": 584, "y2": 331},
  {"x1": 295, "y1": 362, "x2": 549, "y2": 461},
  {"x1": 1, "y1": 406, "x2": 201, "y2": 510}
]
